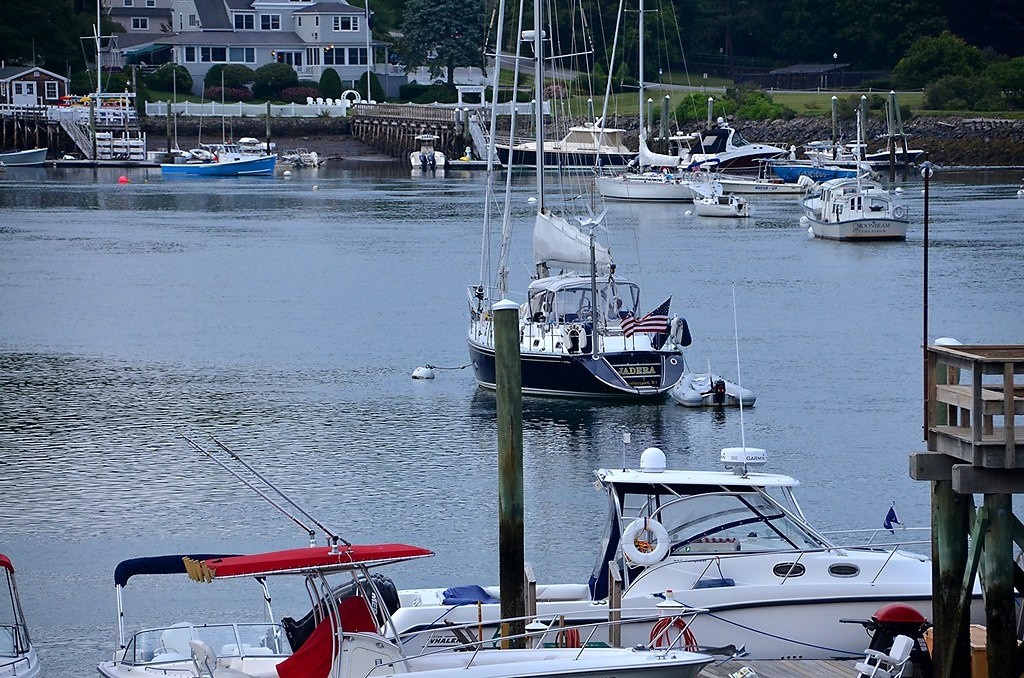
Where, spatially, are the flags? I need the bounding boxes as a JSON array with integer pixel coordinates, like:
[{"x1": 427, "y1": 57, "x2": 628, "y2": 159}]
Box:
[
  {"x1": 619, "y1": 297, "x2": 671, "y2": 338},
  {"x1": 883, "y1": 507, "x2": 898, "y2": 536}
]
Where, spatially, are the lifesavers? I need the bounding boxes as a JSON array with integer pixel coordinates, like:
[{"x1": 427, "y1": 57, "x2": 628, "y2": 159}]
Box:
[
  {"x1": 671, "y1": 318, "x2": 683, "y2": 345},
  {"x1": 621, "y1": 518, "x2": 670, "y2": 565},
  {"x1": 893, "y1": 204, "x2": 905, "y2": 218},
  {"x1": 563, "y1": 324, "x2": 587, "y2": 349}
]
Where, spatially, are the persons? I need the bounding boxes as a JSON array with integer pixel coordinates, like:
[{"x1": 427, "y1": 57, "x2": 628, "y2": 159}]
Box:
[
  {"x1": 661, "y1": 166, "x2": 667, "y2": 175},
  {"x1": 608, "y1": 299, "x2": 622, "y2": 318},
  {"x1": 692, "y1": 160, "x2": 700, "y2": 172},
  {"x1": 283, "y1": 149, "x2": 323, "y2": 168}
]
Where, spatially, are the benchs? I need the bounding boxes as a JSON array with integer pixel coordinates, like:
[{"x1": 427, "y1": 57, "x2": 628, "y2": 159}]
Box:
[
  {"x1": 222, "y1": 644, "x2": 291, "y2": 678},
  {"x1": 937, "y1": 384, "x2": 1024, "y2": 435}
]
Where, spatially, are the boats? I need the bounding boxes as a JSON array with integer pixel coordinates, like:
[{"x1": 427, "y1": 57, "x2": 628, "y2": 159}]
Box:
[
  {"x1": 667, "y1": 368, "x2": 757, "y2": 409},
  {"x1": 0, "y1": 143, "x2": 49, "y2": 167},
  {"x1": 281, "y1": 148, "x2": 321, "y2": 169},
  {"x1": 0, "y1": 552, "x2": 44, "y2": 678},
  {"x1": 160, "y1": 152, "x2": 277, "y2": 176},
  {"x1": 688, "y1": 171, "x2": 752, "y2": 218},
  {"x1": 799, "y1": 107, "x2": 910, "y2": 240},
  {"x1": 402, "y1": 445, "x2": 1024, "y2": 662},
  {"x1": 96, "y1": 432, "x2": 716, "y2": 677},
  {"x1": 677, "y1": 89, "x2": 934, "y2": 194}
]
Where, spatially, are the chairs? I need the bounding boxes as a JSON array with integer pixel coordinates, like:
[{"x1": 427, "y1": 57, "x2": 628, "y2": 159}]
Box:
[
  {"x1": 855, "y1": 634, "x2": 915, "y2": 678},
  {"x1": 189, "y1": 639, "x2": 218, "y2": 678}
]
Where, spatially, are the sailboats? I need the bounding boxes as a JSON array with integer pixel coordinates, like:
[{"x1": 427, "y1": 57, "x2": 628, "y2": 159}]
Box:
[
  {"x1": 467, "y1": 0, "x2": 693, "y2": 404},
  {"x1": 590, "y1": 1, "x2": 724, "y2": 203},
  {"x1": 148, "y1": 68, "x2": 276, "y2": 166}
]
